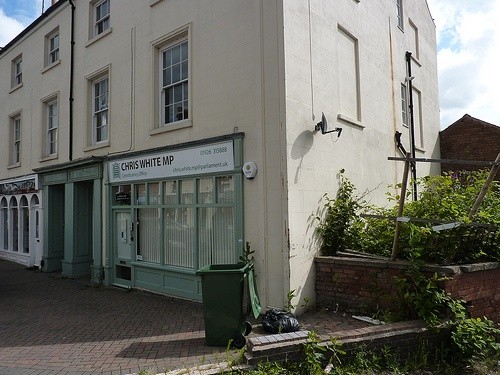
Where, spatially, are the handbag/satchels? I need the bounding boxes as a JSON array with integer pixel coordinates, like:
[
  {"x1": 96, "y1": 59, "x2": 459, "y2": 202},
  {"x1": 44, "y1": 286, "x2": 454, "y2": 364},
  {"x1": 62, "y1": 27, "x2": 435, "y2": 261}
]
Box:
[{"x1": 262, "y1": 306, "x2": 300, "y2": 334}]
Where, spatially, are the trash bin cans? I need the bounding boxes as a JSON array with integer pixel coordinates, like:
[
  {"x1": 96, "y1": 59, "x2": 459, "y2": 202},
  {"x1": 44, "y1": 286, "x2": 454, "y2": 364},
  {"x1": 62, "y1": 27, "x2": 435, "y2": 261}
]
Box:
[{"x1": 195, "y1": 261, "x2": 262, "y2": 348}]
